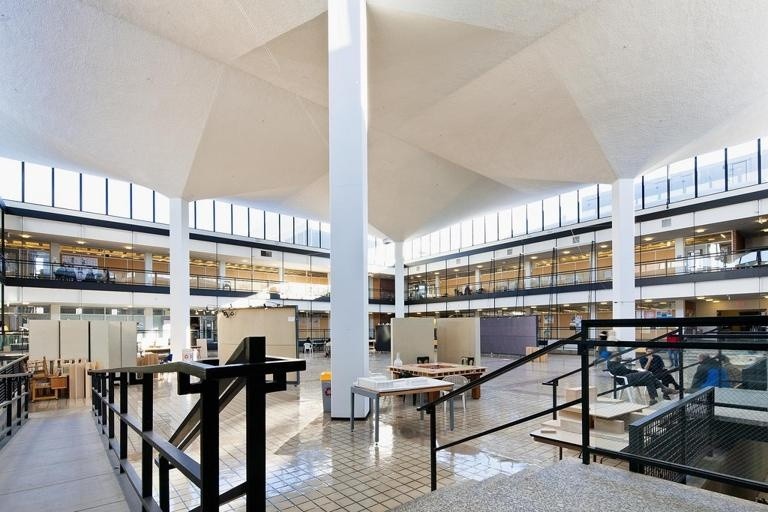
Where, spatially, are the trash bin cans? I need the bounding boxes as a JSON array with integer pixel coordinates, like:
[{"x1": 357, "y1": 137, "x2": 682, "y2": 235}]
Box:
[{"x1": 320, "y1": 371, "x2": 332, "y2": 413}]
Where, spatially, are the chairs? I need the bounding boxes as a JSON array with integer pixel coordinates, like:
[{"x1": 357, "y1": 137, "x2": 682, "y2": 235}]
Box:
[
  {"x1": 416, "y1": 349, "x2": 475, "y2": 413},
  {"x1": 304, "y1": 343, "x2": 313, "y2": 354},
  {"x1": 608, "y1": 369, "x2": 649, "y2": 406},
  {"x1": 24, "y1": 355, "x2": 69, "y2": 402}
]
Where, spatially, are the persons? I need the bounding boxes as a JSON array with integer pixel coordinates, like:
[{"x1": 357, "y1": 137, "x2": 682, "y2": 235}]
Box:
[
  {"x1": 716, "y1": 355, "x2": 742, "y2": 388},
  {"x1": 318, "y1": 338, "x2": 325, "y2": 351},
  {"x1": 640, "y1": 346, "x2": 686, "y2": 400},
  {"x1": 607, "y1": 352, "x2": 678, "y2": 406},
  {"x1": 303, "y1": 338, "x2": 312, "y2": 353},
  {"x1": 666, "y1": 329, "x2": 680, "y2": 368},
  {"x1": 464, "y1": 284, "x2": 472, "y2": 295},
  {"x1": 596, "y1": 331, "x2": 607, "y2": 355},
  {"x1": 688, "y1": 353, "x2": 731, "y2": 395}
]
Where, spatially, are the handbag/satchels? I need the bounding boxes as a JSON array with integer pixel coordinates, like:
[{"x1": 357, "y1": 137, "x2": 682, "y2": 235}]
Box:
[{"x1": 49, "y1": 368, "x2": 68, "y2": 390}]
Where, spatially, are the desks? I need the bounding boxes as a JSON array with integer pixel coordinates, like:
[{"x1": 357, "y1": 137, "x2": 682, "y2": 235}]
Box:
[
  {"x1": 388, "y1": 361, "x2": 488, "y2": 406},
  {"x1": 350, "y1": 376, "x2": 454, "y2": 442}
]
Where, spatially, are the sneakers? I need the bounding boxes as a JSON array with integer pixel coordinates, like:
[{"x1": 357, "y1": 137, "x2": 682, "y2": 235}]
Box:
[
  {"x1": 667, "y1": 363, "x2": 679, "y2": 369},
  {"x1": 648, "y1": 385, "x2": 686, "y2": 405}
]
